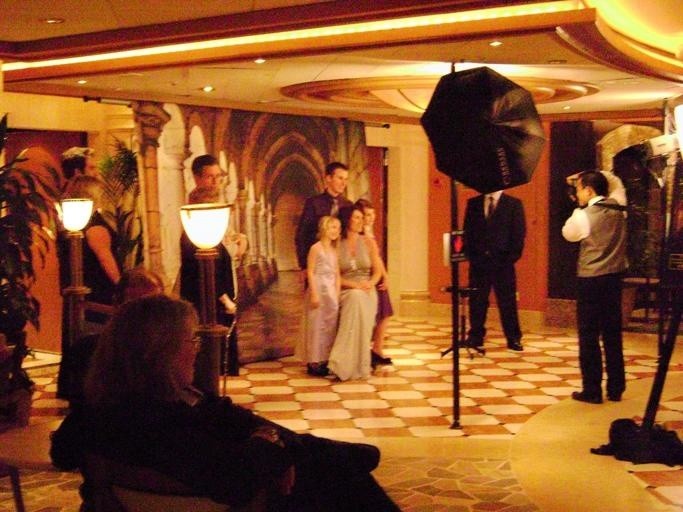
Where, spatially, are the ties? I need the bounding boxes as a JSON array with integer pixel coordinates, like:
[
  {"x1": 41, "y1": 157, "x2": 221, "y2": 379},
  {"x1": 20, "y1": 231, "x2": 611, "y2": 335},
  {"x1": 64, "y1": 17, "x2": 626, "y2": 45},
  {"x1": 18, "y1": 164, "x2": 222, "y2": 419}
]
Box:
[
  {"x1": 489, "y1": 197, "x2": 495, "y2": 216},
  {"x1": 330, "y1": 199, "x2": 339, "y2": 217}
]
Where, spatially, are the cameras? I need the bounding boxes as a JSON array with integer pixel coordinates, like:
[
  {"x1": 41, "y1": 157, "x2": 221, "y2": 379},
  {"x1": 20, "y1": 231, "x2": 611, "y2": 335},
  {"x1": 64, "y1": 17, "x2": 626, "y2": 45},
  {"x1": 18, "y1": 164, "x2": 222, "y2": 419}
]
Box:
[{"x1": 568, "y1": 177, "x2": 577, "y2": 199}]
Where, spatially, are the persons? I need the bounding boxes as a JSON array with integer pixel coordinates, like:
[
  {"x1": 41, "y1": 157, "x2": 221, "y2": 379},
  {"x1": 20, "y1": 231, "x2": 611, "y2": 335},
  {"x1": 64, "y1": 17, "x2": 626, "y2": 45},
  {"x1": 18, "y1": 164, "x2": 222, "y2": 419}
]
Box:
[
  {"x1": 354, "y1": 198, "x2": 395, "y2": 366},
  {"x1": 115, "y1": 270, "x2": 166, "y2": 308},
  {"x1": 294, "y1": 161, "x2": 356, "y2": 293},
  {"x1": 56, "y1": 146, "x2": 126, "y2": 400},
  {"x1": 299, "y1": 214, "x2": 344, "y2": 379},
  {"x1": 173, "y1": 154, "x2": 250, "y2": 376},
  {"x1": 560, "y1": 167, "x2": 629, "y2": 403},
  {"x1": 457, "y1": 189, "x2": 526, "y2": 353},
  {"x1": 47, "y1": 295, "x2": 402, "y2": 512},
  {"x1": 326, "y1": 205, "x2": 386, "y2": 380}
]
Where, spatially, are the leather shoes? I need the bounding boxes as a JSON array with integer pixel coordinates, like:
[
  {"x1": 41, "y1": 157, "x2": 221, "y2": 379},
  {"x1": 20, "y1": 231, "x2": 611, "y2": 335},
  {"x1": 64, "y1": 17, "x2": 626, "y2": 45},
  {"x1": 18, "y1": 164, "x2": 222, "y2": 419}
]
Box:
[
  {"x1": 607, "y1": 391, "x2": 621, "y2": 401},
  {"x1": 572, "y1": 392, "x2": 602, "y2": 403},
  {"x1": 372, "y1": 350, "x2": 391, "y2": 363},
  {"x1": 508, "y1": 343, "x2": 522, "y2": 351},
  {"x1": 465, "y1": 336, "x2": 483, "y2": 346}
]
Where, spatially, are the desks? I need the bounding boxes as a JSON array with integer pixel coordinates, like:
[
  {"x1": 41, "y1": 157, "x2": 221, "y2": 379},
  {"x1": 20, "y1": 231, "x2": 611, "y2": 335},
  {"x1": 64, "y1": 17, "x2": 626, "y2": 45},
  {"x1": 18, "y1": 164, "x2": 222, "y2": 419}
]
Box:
[{"x1": 0, "y1": 416, "x2": 79, "y2": 512}]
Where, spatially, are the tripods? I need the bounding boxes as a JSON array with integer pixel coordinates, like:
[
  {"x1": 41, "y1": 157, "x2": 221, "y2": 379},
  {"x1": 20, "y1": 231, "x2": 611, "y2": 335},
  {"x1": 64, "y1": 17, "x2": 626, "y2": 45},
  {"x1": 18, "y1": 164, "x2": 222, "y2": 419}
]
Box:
[{"x1": 439, "y1": 316, "x2": 487, "y2": 359}]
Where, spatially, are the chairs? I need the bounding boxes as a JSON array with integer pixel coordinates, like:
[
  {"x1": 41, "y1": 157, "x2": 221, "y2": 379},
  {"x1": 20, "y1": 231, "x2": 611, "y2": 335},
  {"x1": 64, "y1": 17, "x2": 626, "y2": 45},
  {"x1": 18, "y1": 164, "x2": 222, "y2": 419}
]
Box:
[{"x1": 111, "y1": 483, "x2": 235, "y2": 512}]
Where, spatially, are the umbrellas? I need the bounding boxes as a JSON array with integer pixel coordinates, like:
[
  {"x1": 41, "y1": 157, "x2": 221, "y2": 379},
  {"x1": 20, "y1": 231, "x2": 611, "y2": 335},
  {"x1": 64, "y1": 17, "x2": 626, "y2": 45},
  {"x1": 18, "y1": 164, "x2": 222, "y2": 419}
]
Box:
[{"x1": 420, "y1": 66, "x2": 546, "y2": 198}]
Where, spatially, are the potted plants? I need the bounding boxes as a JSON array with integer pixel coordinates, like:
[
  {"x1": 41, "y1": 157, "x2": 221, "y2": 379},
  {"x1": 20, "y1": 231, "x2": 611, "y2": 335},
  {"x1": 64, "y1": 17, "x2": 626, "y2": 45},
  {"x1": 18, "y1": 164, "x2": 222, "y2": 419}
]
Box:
[{"x1": 0, "y1": 111, "x2": 67, "y2": 435}]
